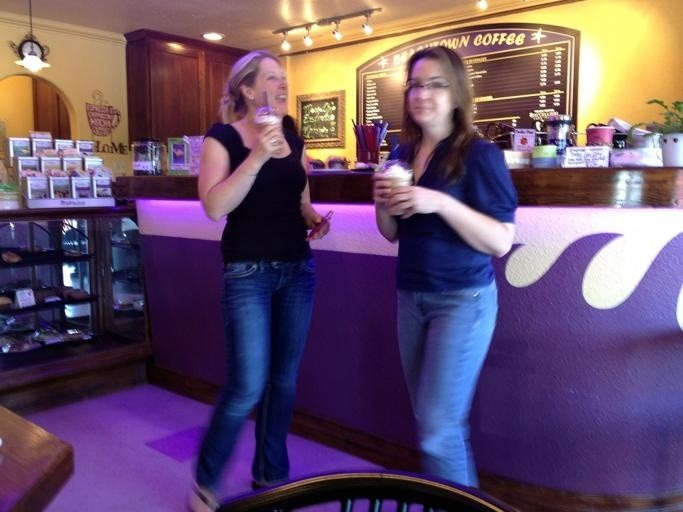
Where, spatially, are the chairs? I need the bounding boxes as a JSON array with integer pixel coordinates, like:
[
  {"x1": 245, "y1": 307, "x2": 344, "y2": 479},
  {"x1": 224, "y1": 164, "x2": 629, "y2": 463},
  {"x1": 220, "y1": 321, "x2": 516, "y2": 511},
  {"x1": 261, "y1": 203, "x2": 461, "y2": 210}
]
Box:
[{"x1": 215, "y1": 467, "x2": 520, "y2": 512}]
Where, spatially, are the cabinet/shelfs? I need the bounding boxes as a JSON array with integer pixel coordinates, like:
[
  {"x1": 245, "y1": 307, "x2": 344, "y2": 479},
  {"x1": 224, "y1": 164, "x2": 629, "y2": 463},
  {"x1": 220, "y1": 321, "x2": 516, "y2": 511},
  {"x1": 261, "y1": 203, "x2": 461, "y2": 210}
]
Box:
[{"x1": 1, "y1": 206, "x2": 151, "y2": 414}]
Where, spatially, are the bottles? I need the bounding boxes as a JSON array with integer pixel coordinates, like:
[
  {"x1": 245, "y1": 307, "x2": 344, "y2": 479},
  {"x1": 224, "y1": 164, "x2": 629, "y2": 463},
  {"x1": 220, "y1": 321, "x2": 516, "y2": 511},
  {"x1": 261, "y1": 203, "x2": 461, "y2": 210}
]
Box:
[{"x1": 130, "y1": 134, "x2": 167, "y2": 175}]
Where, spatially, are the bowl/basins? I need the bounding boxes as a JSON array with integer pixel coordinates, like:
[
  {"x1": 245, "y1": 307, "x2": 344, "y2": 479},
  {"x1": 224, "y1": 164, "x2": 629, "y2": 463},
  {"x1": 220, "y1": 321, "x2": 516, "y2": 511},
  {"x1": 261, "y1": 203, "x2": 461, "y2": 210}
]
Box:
[{"x1": 327, "y1": 160, "x2": 348, "y2": 169}]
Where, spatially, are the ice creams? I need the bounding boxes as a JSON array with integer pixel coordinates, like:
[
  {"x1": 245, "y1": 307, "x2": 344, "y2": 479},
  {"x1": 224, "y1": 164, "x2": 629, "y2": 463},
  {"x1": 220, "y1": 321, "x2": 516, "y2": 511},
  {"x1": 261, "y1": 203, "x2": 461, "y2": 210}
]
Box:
[
  {"x1": 378, "y1": 159, "x2": 414, "y2": 215},
  {"x1": 254, "y1": 107, "x2": 292, "y2": 159}
]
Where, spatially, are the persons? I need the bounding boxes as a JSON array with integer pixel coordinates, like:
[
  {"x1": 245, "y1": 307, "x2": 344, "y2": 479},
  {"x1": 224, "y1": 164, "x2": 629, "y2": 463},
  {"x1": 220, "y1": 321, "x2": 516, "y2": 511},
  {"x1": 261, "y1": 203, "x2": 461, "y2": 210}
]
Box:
[
  {"x1": 184, "y1": 50, "x2": 331, "y2": 512},
  {"x1": 372, "y1": 45, "x2": 518, "y2": 490}
]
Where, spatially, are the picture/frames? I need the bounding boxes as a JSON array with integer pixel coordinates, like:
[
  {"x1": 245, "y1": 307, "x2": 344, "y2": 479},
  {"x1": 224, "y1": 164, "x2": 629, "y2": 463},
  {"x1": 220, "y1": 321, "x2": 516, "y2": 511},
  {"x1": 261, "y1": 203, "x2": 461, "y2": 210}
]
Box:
[{"x1": 296, "y1": 89, "x2": 345, "y2": 148}]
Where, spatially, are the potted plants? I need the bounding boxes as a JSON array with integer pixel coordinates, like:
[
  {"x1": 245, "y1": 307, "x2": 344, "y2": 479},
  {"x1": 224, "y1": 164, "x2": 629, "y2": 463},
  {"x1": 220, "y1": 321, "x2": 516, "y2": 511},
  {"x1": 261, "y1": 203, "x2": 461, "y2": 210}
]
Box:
[{"x1": 627, "y1": 99, "x2": 683, "y2": 167}]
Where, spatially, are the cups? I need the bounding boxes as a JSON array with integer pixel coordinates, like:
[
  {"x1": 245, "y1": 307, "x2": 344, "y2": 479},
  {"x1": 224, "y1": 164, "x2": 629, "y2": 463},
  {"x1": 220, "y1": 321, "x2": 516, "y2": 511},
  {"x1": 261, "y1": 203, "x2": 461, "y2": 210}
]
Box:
[
  {"x1": 484, "y1": 122, "x2": 683, "y2": 168},
  {"x1": 378, "y1": 152, "x2": 391, "y2": 163},
  {"x1": 357, "y1": 151, "x2": 378, "y2": 165},
  {"x1": 255, "y1": 116, "x2": 285, "y2": 155},
  {"x1": 377, "y1": 173, "x2": 411, "y2": 215}
]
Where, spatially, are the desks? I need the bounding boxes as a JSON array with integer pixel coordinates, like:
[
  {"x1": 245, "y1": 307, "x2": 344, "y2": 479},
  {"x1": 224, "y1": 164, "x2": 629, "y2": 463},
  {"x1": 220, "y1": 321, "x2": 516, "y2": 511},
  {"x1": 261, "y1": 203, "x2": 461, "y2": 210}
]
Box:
[{"x1": 0, "y1": 402, "x2": 73, "y2": 512}]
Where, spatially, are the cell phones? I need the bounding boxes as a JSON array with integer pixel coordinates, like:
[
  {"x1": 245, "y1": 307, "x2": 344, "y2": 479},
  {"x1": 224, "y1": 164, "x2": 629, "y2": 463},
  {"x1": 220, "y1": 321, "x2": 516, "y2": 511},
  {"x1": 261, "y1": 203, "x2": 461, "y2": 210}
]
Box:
[{"x1": 309, "y1": 210, "x2": 334, "y2": 239}]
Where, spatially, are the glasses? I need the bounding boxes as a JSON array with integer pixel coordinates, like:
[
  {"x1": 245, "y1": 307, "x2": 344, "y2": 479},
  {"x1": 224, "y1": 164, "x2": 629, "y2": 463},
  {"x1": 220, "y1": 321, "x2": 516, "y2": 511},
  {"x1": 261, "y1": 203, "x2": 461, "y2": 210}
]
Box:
[{"x1": 406, "y1": 80, "x2": 449, "y2": 92}]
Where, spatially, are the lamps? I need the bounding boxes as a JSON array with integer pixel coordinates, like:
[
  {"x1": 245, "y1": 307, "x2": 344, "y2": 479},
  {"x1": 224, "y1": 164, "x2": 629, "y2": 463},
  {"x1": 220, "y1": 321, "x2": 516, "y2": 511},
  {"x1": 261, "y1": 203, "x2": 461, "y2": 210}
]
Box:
[
  {"x1": 316, "y1": 8, "x2": 381, "y2": 41},
  {"x1": 14, "y1": 0, "x2": 51, "y2": 72},
  {"x1": 272, "y1": 22, "x2": 316, "y2": 51}
]
